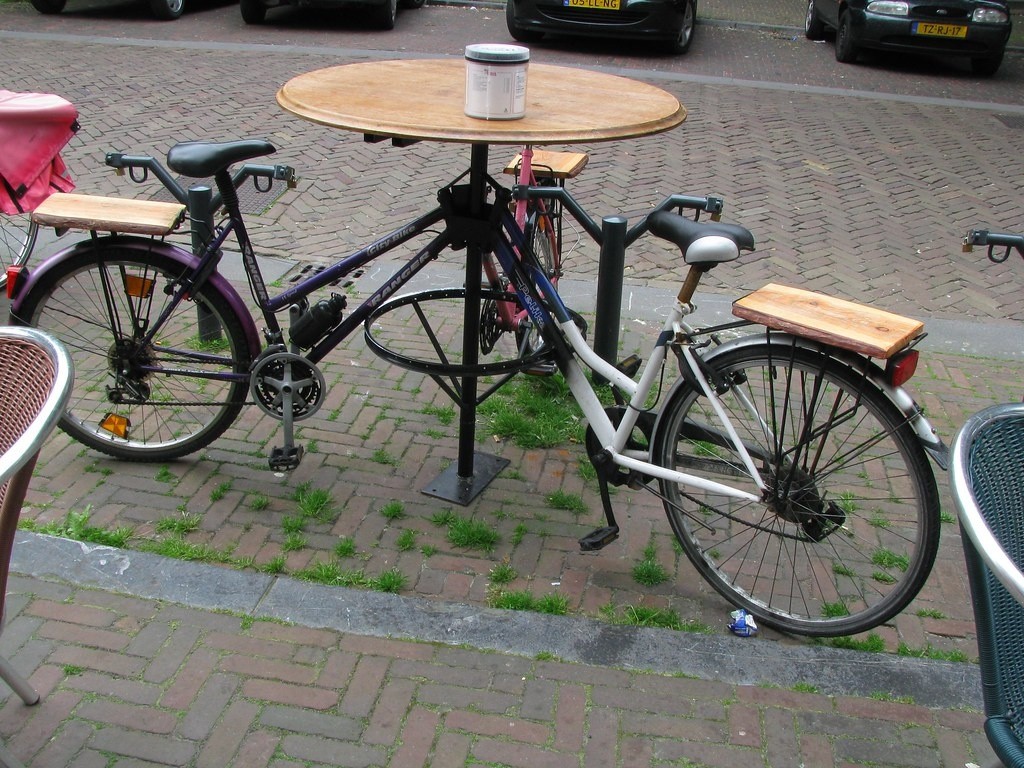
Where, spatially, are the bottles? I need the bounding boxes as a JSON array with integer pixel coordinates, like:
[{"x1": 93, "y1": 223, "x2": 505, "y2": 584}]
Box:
[{"x1": 289, "y1": 292, "x2": 347, "y2": 348}]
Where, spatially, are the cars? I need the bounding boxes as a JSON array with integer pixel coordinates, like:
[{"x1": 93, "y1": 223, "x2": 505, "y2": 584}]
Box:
[
  {"x1": 504, "y1": 0, "x2": 699, "y2": 57},
  {"x1": 805, "y1": 0, "x2": 1012, "y2": 79},
  {"x1": 240, "y1": 0, "x2": 426, "y2": 31}
]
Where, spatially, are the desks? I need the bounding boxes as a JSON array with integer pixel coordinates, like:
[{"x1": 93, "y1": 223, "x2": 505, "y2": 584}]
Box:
[{"x1": 275, "y1": 59, "x2": 687, "y2": 507}]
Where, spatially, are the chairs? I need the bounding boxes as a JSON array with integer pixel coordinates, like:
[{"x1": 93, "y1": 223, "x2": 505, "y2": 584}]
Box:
[
  {"x1": 0, "y1": 325, "x2": 76, "y2": 707},
  {"x1": 951, "y1": 403, "x2": 1024, "y2": 768}
]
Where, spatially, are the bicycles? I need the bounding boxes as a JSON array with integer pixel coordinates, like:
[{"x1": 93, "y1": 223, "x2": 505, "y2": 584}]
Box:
[
  {"x1": 6, "y1": 138, "x2": 453, "y2": 466},
  {"x1": 489, "y1": 184, "x2": 950, "y2": 640},
  {"x1": 476, "y1": 135, "x2": 590, "y2": 377}
]
